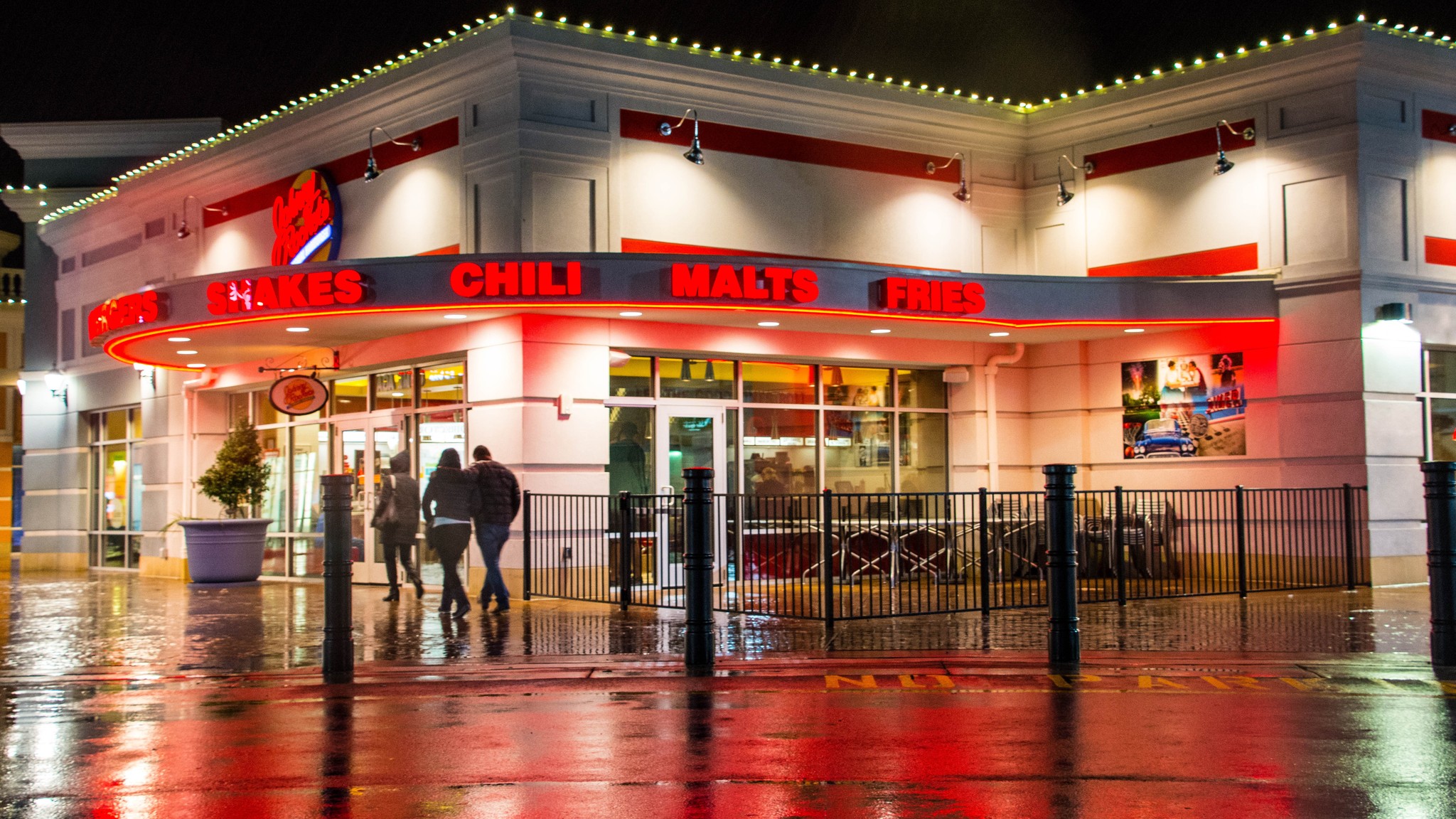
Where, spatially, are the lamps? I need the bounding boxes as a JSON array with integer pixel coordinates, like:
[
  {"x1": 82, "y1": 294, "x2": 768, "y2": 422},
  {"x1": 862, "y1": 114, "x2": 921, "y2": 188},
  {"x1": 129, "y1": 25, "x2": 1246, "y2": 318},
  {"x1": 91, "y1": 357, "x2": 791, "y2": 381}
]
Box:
[
  {"x1": 364, "y1": 127, "x2": 423, "y2": 185},
  {"x1": 176, "y1": 195, "x2": 230, "y2": 239},
  {"x1": 1378, "y1": 302, "x2": 1417, "y2": 325},
  {"x1": 704, "y1": 361, "x2": 715, "y2": 382},
  {"x1": 1213, "y1": 118, "x2": 1254, "y2": 176},
  {"x1": 43, "y1": 361, "x2": 69, "y2": 409},
  {"x1": 1056, "y1": 154, "x2": 1094, "y2": 206},
  {"x1": 808, "y1": 365, "x2": 816, "y2": 387},
  {"x1": 926, "y1": 151, "x2": 972, "y2": 205},
  {"x1": 660, "y1": 108, "x2": 706, "y2": 165},
  {"x1": 681, "y1": 358, "x2": 691, "y2": 381},
  {"x1": 423, "y1": 387, "x2": 462, "y2": 423},
  {"x1": 831, "y1": 367, "x2": 843, "y2": 387}
]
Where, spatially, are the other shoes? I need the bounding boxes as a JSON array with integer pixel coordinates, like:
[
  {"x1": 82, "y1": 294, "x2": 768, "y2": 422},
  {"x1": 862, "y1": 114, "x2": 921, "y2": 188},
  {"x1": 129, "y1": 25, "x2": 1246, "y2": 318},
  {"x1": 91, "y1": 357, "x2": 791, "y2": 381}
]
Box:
[
  {"x1": 451, "y1": 601, "x2": 471, "y2": 619},
  {"x1": 438, "y1": 605, "x2": 451, "y2": 613},
  {"x1": 481, "y1": 594, "x2": 492, "y2": 608},
  {"x1": 491, "y1": 599, "x2": 510, "y2": 613}
]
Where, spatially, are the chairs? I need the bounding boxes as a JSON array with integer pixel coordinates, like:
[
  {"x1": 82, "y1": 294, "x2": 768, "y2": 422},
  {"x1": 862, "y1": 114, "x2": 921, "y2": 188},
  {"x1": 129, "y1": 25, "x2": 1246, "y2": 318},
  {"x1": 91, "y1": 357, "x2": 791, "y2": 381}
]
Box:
[{"x1": 986, "y1": 496, "x2": 1176, "y2": 584}]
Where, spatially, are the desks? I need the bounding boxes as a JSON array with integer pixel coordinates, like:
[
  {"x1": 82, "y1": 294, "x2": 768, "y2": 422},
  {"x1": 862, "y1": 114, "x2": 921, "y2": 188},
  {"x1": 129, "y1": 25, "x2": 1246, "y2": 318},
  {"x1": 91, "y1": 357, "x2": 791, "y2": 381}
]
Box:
[{"x1": 802, "y1": 519, "x2": 1041, "y2": 586}]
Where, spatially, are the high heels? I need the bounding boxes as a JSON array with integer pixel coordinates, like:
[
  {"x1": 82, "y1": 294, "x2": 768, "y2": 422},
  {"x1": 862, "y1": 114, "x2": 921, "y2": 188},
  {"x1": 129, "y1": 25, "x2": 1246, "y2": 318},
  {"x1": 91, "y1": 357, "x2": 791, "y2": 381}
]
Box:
[
  {"x1": 413, "y1": 577, "x2": 424, "y2": 598},
  {"x1": 383, "y1": 588, "x2": 400, "y2": 601}
]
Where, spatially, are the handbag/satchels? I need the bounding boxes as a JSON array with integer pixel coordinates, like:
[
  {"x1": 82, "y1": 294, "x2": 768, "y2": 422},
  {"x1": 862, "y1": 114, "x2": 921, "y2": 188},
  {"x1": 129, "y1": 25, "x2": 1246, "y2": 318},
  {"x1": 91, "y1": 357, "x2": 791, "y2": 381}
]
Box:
[
  {"x1": 374, "y1": 474, "x2": 401, "y2": 530},
  {"x1": 424, "y1": 517, "x2": 434, "y2": 549}
]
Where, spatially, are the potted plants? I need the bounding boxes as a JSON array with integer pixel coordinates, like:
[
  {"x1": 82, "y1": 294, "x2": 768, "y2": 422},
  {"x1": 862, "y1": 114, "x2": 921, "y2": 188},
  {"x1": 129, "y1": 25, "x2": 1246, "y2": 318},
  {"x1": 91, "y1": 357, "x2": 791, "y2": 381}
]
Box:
[{"x1": 176, "y1": 405, "x2": 276, "y2": 583}]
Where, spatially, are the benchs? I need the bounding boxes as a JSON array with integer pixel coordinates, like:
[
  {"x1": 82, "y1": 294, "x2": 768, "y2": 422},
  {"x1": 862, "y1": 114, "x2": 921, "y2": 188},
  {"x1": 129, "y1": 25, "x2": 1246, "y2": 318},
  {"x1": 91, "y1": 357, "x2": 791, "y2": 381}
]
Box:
[{"x1": 262, "y1": 547, "x2": 360, "y2": 574}]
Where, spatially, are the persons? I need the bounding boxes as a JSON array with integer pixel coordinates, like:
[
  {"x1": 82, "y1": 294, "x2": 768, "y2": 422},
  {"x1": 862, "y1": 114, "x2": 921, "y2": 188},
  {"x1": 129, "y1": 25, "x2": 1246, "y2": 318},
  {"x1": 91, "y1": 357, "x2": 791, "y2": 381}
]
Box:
[
  {"x1": 437, "y1": 445, "x2": 520, "y2": 614},
  {"x1": 421, "y1": 448, "x2": 470, "y2": 619},
  {"x1": 370, "y1": 448, "x2": 424, "y2": 602},
  {"x1": 1161, "y1": 360, "x2": 1236, "y2": 399}
]
